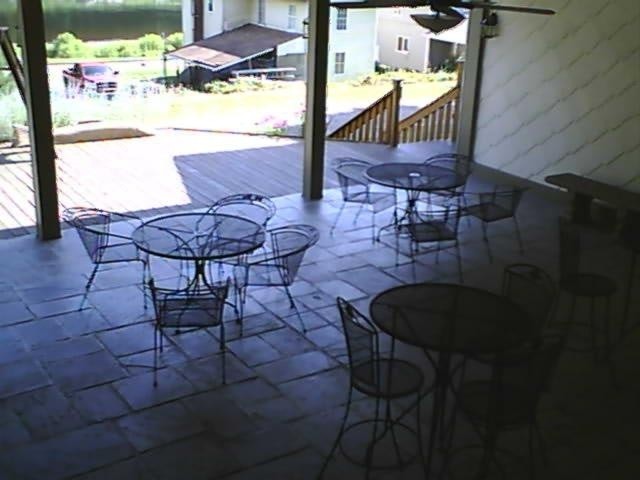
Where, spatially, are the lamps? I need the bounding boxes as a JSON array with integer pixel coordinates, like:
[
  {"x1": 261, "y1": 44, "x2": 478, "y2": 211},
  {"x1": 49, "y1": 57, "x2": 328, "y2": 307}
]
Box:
[
  {"x1": 480, "y1": 12, "x2": 498, "y2": 39},
  {"x1": 410, "y1": 4, "x2": 465, "y2": 33}
]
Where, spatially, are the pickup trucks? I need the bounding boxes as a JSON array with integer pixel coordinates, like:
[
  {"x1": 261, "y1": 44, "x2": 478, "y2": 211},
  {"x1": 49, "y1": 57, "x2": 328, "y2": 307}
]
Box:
[{"x1": 59, "y1": 61, "x2": 125, "y2": 100}]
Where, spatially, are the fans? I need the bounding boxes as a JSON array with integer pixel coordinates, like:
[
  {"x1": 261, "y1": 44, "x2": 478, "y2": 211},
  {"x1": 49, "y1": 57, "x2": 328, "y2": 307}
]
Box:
[{"x1": 331, "y1": 0, "x2": 556, "y2": 16}]
